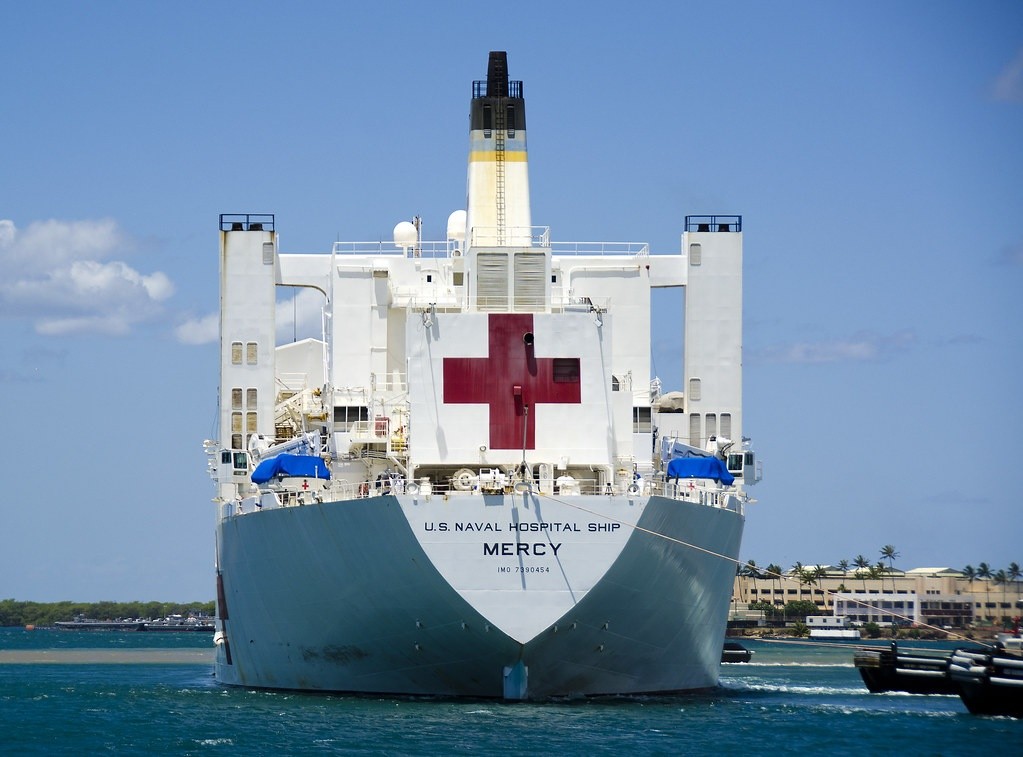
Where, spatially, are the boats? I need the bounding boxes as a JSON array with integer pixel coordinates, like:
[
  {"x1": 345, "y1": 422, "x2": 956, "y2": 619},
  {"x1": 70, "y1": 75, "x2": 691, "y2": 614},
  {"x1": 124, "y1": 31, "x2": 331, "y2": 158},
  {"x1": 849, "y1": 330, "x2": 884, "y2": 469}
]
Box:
[
  {"x1": 203, "y1": 50, "x2": 765, "y2": 703},
  {"x1": 253, "y1": 456, "x2": 331, "y2": 494},
  {"x1": 26, "y1": 625, "x2": 35, "y2": 630},
  {"x1": 949, "y1": 643, "x2": 1022, "y2": 719},
  {"x1": 722, "y1": 642, "x2": 755, "y2": 663},
  {"x1": 851, "y1": 639, "x2": 958, "y2": 695},
  {"x1": 667, "y1": 456, "x2": 736, "y2": 501},
  {"x1": 144, "y1": 614, "x2": 215, "y2": 632},
  {"x1": 809, "y1": 625, "x2": 862, "y2": 641},
  {"x1": 55, "y1": 615, "x2": 149, "y2": 631}
]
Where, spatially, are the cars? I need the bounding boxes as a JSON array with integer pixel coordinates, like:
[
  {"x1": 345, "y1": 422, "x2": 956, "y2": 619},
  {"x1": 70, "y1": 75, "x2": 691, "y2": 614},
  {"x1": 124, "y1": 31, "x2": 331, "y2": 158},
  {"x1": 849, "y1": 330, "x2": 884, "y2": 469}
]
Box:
[{"x1": 892, "y1": 619, "x2": 910, "y2": 626}]
